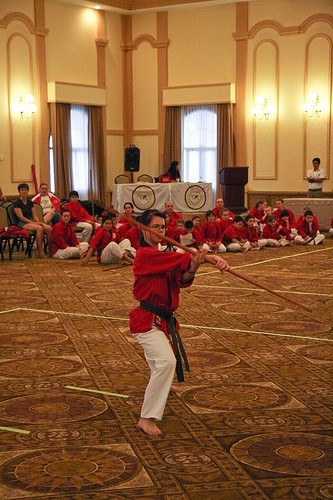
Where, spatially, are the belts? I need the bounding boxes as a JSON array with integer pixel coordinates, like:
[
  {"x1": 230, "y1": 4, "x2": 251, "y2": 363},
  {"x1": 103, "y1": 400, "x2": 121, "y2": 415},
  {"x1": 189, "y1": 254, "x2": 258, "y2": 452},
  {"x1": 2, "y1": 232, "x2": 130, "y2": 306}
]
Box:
[{"x1": 309, "y1": 189, "x2": 321, "y2": 191}]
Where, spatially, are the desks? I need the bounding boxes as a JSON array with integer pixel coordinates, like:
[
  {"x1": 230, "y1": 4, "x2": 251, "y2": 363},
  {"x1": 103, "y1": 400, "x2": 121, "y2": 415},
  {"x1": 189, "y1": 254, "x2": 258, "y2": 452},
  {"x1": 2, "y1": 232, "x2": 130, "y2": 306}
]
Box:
[
  {"x1": 106, "y1": 181, "x2": 215, "y2": 215},
  {"x1": 281, "y1": 198, "x2": 333, "y2": 231}
]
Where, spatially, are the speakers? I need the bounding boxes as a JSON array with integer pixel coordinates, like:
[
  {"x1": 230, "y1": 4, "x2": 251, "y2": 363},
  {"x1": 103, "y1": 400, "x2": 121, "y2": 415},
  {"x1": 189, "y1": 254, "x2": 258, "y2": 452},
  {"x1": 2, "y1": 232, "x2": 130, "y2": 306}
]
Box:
[{"x1": 125, "y1": 147, "x2": 141, "y2": 173}]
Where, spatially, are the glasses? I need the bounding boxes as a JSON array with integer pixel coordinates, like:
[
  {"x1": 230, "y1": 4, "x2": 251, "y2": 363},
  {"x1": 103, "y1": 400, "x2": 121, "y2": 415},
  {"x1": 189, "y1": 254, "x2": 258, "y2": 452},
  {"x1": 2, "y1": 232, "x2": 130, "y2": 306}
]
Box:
[{"x1": 150, "y1": 225, "x2": 168, "y2": 231}]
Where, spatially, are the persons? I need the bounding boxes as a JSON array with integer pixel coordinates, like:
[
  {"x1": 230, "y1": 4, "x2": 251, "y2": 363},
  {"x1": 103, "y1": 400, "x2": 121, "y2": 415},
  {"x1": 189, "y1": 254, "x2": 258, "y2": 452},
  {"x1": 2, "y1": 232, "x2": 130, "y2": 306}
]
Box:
[
  {"x1": 13, "y1": 183, "x2": 53, "y2": 258},
  {"x1": 130, "y1": 208, "x2": 229, "y2": 434},
  {"x1": 82, "y1": 199, "x2": 325, "y2": 265},
  {"x1": 163, "y1": 161, "x2": 182, "y2": 182},
  {"x1": 31, "y1": 183, "x2": 62, "y2": 225},
  {"x1": 0, "y1": 188, "x2": 6, "y2": 201},
  {"x1": 304, "y1": 158, "x2": 325, "y2": 197},
  {"x1": 66, "y1": 190, "x2": 95, "y2": 242},
  {"x1": 47, "y1": 209, "x2": 89, "y2": 260}
]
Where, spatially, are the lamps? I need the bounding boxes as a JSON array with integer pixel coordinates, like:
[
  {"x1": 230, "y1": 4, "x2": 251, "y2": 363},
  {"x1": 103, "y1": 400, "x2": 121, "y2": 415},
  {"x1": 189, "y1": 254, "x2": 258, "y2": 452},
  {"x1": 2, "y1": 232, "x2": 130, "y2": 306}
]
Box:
[
  {"x1": 249, "y1": 96, "x2": 275, "y2": 122},
  {"x1": 13, "y1": 91, "x2": 37, "y2": 120},
  {"x1": 301, "y1": 91, "x2": 327, "y2": 119}
]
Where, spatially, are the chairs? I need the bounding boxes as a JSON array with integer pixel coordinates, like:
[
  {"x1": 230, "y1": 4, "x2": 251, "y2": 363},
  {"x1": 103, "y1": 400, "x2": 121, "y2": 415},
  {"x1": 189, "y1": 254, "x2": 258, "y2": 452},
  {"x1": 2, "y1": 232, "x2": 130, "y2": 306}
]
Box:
[
  {"x1": 60, "y1": 201, "x2": 83, "y2": 233},
  {"x1": 0, "y1": 199, "x2": 49, "y2": 261},
  {"x1": 114, "y1": 174, "x2": 131, "y2": 184},
  {"x1": 138, "y1": 173, "x2": 153, "y2": 183}
]
